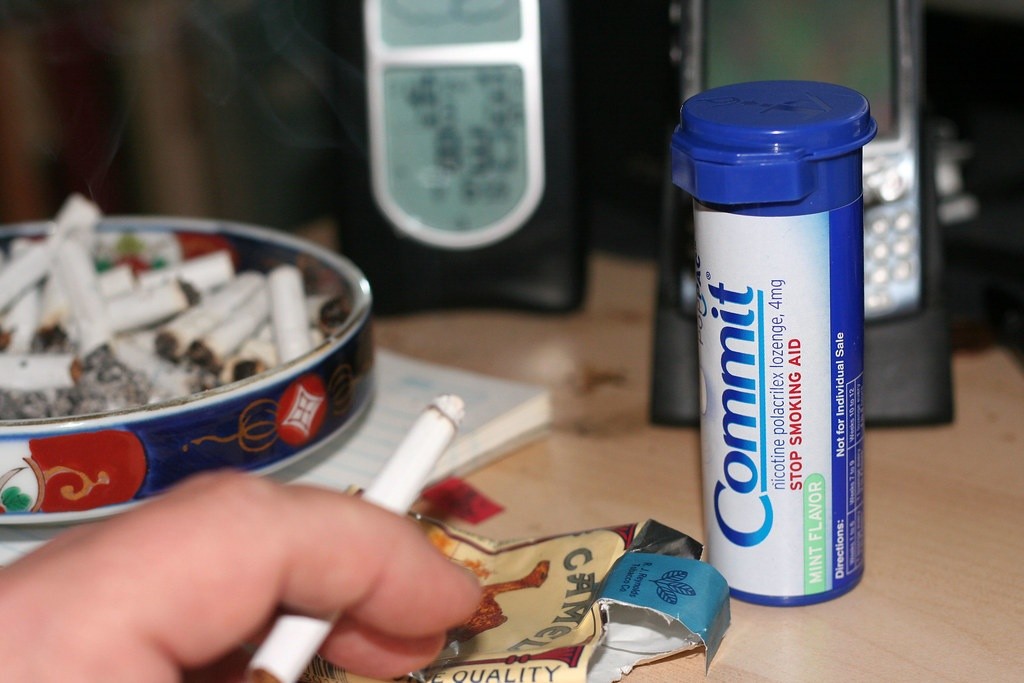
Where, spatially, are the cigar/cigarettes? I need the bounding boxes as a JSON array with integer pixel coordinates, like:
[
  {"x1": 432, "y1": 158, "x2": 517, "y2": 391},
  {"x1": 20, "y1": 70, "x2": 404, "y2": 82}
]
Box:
[
  {"x1": 0, "y1": 194, "x2": 351, "y2": 398},
  {"x1": 240, "y1": 393, "x2": 469, "y2": 682}
]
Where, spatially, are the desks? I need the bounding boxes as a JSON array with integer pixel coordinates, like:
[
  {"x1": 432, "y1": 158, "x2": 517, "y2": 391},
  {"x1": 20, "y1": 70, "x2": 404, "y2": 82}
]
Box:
[{"x1": 368, "y1": 253, "x2": 1024, "y2": 681}]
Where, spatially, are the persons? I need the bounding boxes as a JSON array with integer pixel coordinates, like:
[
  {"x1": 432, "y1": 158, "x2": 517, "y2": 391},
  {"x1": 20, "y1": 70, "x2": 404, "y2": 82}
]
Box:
[{"x1": 0, "y1": 466, "x2": 486, "y2": 683}]
